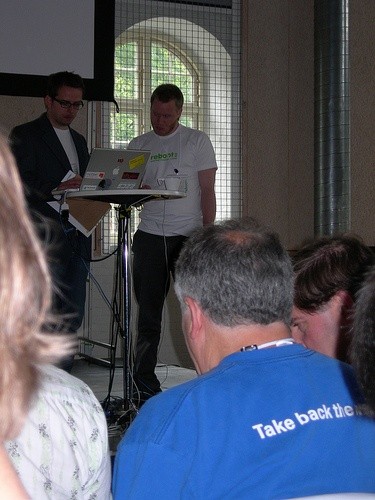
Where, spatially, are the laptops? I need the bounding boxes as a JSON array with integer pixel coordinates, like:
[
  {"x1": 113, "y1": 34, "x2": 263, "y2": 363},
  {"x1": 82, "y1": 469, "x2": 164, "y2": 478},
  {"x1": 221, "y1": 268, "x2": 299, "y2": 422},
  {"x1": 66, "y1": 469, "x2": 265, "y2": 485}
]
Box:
[{"x1": 51, "y1": 148, "x2": 152, "y2": 195}]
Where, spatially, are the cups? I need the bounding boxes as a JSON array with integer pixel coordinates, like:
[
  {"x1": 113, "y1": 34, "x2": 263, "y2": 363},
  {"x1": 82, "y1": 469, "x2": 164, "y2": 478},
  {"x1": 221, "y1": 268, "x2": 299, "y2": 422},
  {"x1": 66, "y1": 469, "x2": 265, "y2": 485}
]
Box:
[{"x1": 166, "y1": 175, "x2": 180, "y2": 190}]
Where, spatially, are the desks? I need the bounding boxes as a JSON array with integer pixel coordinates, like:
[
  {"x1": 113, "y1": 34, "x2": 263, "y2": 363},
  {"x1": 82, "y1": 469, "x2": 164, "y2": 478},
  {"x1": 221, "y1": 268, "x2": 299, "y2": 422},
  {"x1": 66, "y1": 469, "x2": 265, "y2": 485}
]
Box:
[{"x1": 61, "y1": 189, "x2": 187, "y2": 438}]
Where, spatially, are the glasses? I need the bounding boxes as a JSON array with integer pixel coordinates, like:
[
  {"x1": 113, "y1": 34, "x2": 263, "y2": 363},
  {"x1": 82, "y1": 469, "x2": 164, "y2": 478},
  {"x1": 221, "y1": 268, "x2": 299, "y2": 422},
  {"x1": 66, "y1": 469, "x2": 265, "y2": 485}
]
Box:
[{"x1": 53, "y1": 98, "x2": 84, "y2": 111}]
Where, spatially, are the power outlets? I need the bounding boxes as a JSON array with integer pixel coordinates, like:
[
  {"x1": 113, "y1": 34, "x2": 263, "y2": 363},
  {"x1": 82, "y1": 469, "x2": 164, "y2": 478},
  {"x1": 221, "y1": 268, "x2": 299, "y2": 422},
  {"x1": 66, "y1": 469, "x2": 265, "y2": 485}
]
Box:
[{"x1": 101, "y1": 409, "x2": 136, "y2": 442}]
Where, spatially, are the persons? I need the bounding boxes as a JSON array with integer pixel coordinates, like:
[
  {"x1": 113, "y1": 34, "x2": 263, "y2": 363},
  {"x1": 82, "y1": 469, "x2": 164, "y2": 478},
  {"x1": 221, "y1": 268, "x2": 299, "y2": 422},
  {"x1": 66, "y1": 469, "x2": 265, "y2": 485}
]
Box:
[
  {"x1": 0, "y1": 135, "x2": 375, "y2": 500},
  {"x1": 126, "y1": 84, "x2": 219, "y2": 404},
  {"x1": 10, "y1": 70, "x2": 92, "y2": 372}
]
